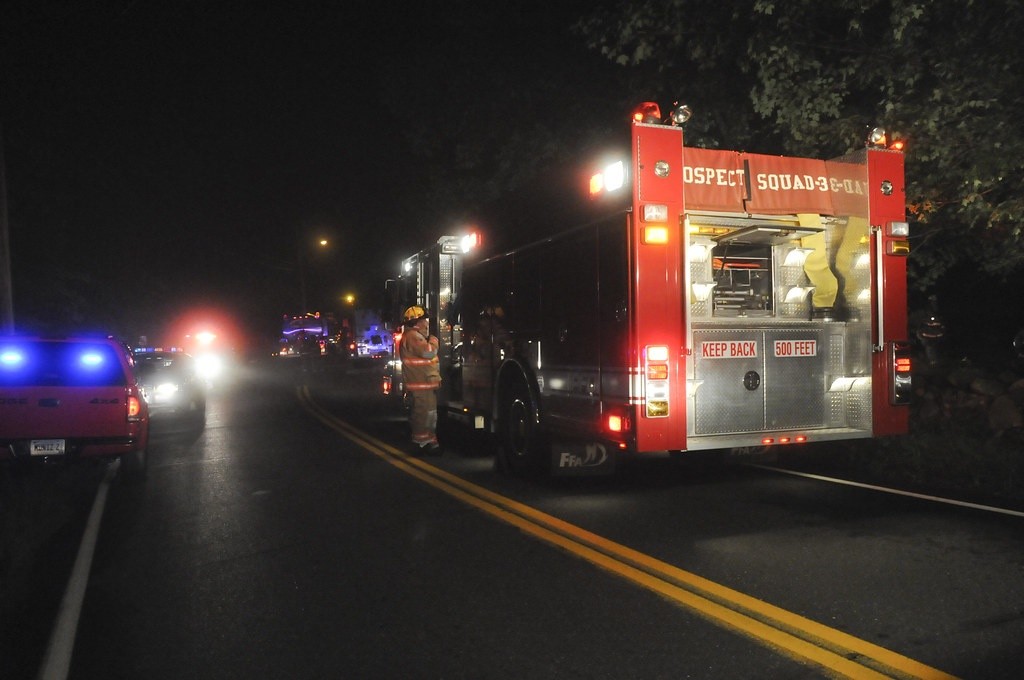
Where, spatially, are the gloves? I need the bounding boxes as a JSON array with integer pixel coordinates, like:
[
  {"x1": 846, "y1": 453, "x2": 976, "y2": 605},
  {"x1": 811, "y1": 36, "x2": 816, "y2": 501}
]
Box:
[{"x1": 429, "y1": 335, "x2": 440, "y2": 350}]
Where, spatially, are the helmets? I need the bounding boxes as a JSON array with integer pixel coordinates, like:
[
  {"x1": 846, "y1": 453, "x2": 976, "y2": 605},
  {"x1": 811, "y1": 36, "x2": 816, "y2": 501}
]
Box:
[{"x1": 403, "y1": 305, "x2": 428, "y2": 324}]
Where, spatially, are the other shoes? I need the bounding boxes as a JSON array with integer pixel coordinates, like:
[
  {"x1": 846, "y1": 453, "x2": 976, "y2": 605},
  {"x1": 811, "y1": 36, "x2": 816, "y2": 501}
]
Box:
[{"x1": 410, "y1": 441, "x2": 444, "y2": 456}]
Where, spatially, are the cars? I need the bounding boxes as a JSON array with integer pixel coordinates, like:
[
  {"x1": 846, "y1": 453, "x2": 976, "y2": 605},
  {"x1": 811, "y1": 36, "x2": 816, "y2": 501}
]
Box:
[
  {"x1": 0, "y1": 325, "x2": 152, "y2": 483},
  {"x1": 120, "y1": 312, "x2": 402, "y2": 436}
]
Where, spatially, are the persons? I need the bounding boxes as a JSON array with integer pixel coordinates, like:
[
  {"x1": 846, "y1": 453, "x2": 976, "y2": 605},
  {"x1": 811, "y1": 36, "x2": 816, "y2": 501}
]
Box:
[{"x1": 399, "y1": 305, "x2": 444, "y2": 457}]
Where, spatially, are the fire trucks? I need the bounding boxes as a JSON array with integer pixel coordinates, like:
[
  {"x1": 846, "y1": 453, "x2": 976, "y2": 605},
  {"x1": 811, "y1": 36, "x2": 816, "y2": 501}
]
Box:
[{"x1": 382, "y1": 100, "x2": 913, "y2": 493}]
